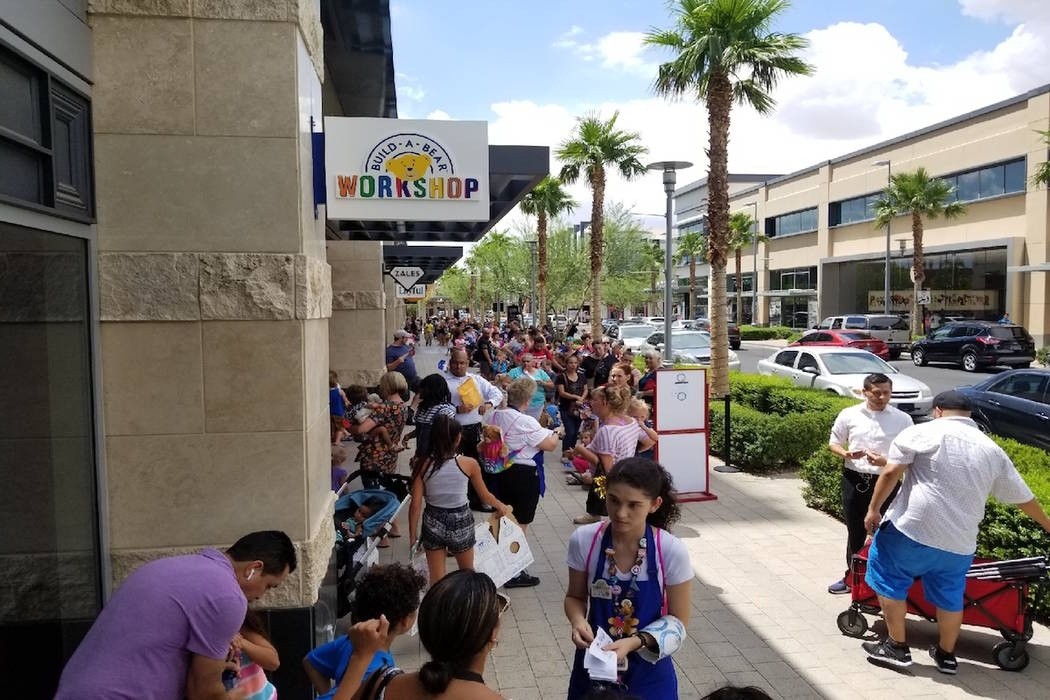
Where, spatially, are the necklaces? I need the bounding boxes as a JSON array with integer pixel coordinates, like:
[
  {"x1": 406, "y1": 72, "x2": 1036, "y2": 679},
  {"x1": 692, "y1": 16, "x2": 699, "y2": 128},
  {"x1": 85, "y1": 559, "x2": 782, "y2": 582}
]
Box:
[{"x1": 605, "y1": 530, "x2": 650, "y2": 637}]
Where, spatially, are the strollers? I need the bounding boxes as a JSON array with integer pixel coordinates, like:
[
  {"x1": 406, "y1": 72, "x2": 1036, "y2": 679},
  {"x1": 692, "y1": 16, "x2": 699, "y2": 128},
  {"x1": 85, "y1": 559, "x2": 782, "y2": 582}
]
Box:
[{"x1": 331, "y1": 467, "x2": 413, "y2": 617}]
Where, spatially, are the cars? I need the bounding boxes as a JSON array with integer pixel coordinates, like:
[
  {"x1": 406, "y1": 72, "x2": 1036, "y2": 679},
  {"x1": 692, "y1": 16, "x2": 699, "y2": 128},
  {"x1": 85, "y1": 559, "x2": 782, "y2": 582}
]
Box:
[
  {"x1": 811, "y1": 315, "x2": 913, "y2": 359},
  {"x1": 756, "y1": 345, "x2": 934, "y2": 419},
  {"x1": 603, "y1": 315, "x2": 744, "y2": 374},
  {"x1": 782, "y1": 329, "x2": 891, "y2": 362},
  {"x1": 487, "y1": 311, "x2": 572, "y2": 332},
  {"x1": 911, "y1": 320, "x2": 1038, "y2": 373},
  {"x1": 953, "y1": 368, "x2": 1050, "y2": 454}
]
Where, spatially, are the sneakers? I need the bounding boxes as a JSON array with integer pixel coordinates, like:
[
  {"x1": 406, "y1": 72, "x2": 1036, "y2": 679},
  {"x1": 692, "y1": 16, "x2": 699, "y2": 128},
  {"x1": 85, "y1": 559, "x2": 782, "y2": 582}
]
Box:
[
  {"x1": 930, "y1": 643, "x2": 958, "y2": 673},
  {"x1": 860, "y1": 640, "x2": 913, "y2": 667},
  {"x1": 504, "y1": 570, "x2": 540, "y2": 588},
  {"x1": 829, "y1": 580, "x2": 850, "y2": 593}
]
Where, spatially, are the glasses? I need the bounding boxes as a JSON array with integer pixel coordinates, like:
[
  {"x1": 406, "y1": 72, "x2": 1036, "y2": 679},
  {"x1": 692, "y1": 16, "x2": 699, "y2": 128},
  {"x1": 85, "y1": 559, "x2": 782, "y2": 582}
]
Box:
[{"x1": 493, "y1": 590, "x2": 512, "y2": 618}]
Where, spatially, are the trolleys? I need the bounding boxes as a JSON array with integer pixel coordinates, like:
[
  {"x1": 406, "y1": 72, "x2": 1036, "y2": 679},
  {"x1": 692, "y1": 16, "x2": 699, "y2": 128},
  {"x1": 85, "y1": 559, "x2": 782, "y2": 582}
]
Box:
[{"x1": 837, "y1": 538, "x2": 1050, "y2": 673}]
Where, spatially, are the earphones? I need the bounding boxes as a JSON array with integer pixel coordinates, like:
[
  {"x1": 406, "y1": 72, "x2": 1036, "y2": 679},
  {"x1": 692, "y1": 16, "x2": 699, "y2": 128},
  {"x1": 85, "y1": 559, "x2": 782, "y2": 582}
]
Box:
[{"x1": 246, "y1": 568, "x2": 255, "y2": 581}]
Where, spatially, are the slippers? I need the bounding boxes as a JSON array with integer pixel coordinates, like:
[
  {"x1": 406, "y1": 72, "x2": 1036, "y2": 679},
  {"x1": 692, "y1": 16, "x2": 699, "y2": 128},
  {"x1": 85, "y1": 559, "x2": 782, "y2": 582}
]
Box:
[
  {"x1": 375, "y1": 545, "x2": 389, "y2": 548},
  {"x1": 388, "y1": 532, "x2": 402, "y2": 538}
]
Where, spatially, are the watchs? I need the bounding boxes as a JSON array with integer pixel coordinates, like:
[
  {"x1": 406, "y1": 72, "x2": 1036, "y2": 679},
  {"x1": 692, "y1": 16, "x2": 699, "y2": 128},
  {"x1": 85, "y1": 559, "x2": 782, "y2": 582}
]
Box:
[
  {"x1": 554, "y1": 430, "x2": 563, "y2": 441},
  {"x1": 630, "y1": 632, "x2": 647, "y2": 655}
]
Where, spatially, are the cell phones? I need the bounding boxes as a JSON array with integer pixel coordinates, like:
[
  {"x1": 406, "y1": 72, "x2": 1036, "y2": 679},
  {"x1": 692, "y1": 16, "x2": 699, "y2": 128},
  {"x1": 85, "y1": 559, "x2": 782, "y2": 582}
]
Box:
[{"x1": 853, "y1": 450, "x2": 863, "y2": 456}]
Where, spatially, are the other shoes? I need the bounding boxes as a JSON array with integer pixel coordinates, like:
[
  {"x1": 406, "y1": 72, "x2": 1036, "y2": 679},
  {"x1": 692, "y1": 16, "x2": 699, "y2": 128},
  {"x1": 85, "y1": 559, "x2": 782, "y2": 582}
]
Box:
[
  {"x1": 573, "y1": 513, "x2": 601, "y2": 524},
  {"x1": 561, "y1": 457, "x2": 570, "y2": 464},
  {"x1": 390, "y1": 444, "x2": 405, "y2": 454}
]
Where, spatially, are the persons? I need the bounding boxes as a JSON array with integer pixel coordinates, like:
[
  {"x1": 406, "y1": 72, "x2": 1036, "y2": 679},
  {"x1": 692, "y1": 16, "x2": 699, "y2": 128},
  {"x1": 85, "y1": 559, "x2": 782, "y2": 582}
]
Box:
[
  {"x1": 829, "y1": 372, "x2": 916, "y2": 594},
  {"x1": 865, "y1": 392, "x2": 1050, "y2": 673},
  {"x1": 48, "y1": 313, "x2": 691, "y2": 700},
  {"x1": 924, "y1": 312, "x2": 942, "y2": 335},
  {"x1": 999, "y1": 313, "x2": 1012, "y2": 323}
]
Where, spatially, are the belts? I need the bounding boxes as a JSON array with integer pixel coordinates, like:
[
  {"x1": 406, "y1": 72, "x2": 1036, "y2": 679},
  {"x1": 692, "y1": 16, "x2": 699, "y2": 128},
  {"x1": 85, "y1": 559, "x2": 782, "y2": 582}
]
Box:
[{"x1": 845, "y1": 469, "x2": 881, "y2": 479}]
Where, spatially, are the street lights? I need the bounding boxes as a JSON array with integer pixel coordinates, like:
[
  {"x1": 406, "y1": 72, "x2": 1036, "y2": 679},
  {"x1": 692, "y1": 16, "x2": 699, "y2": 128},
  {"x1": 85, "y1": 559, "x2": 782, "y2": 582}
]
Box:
[
  {"x1": 872, "y1": 159, "x2": 892, "y2": 314},
  {"x1": 646, "y1": 159, "x2": 695, "y2": 370},
  {"x1": 743, "y1": 201, "x2": 759, "y2": 328},
  {"x1": 526, "y1": 239, "x2": 540, "y2": 329}
]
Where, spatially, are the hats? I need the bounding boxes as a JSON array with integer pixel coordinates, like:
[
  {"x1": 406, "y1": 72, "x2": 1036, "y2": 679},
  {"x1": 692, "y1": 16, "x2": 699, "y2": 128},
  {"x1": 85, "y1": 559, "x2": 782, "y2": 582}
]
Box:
[
  {"x1": 933, "y1": 388, "x2": 973, "y2": 411},
  {"x1": 393, "y1": 329, "x2": 412, "y2": 338}
]
